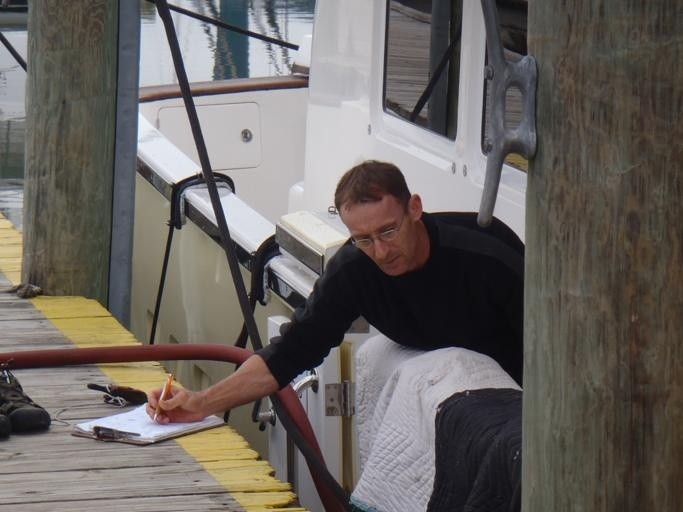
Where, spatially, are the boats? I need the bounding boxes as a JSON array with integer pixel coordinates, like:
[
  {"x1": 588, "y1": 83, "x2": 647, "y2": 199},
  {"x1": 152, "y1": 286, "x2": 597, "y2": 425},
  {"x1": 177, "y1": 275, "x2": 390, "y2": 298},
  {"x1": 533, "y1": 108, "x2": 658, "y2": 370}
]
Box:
[{"x1": 131, "y1": 1, "x2": 532, "y2": 512}]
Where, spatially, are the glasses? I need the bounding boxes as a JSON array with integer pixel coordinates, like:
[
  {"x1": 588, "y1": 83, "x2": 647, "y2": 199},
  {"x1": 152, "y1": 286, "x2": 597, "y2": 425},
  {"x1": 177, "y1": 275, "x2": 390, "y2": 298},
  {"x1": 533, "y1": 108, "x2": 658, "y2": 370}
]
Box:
[{"x1": 349, "y1": 211, "x2": 405, "y2": 250}]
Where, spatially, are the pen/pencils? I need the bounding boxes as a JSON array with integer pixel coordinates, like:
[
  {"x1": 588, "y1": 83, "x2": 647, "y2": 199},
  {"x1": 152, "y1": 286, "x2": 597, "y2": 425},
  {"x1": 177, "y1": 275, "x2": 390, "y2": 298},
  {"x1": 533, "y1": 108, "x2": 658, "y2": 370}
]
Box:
[{"x1": 153, "y1": 373, "x2": 173, "y2": 421}]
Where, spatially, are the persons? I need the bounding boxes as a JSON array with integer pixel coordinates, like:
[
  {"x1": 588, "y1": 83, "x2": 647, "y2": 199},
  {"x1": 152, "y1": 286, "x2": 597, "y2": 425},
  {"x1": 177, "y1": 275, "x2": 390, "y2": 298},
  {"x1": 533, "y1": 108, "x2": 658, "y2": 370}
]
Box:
[{"x1": 146, "y1": 159, "x2": 524, "y2": 425}]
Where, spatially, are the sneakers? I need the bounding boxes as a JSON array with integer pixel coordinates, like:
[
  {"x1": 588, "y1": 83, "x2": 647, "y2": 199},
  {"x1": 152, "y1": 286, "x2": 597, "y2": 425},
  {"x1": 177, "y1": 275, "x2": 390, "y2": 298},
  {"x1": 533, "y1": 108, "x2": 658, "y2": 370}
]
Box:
[{"x1": 0, "y1": 357, "x2": 50, "y2": 438}]
